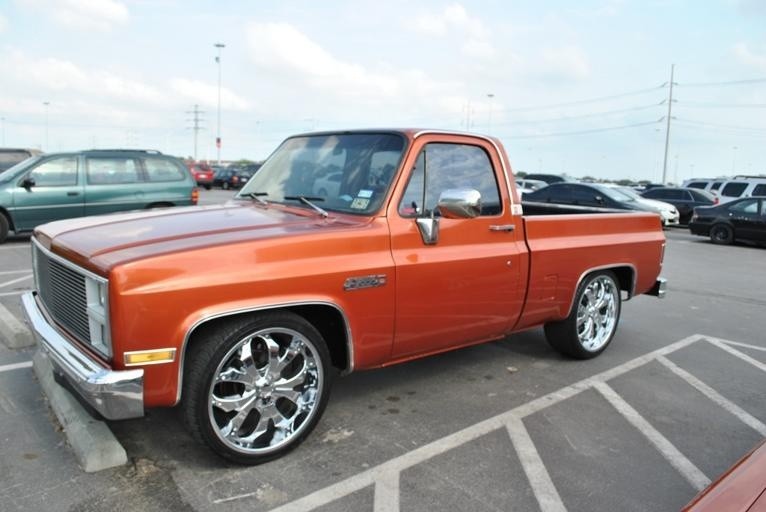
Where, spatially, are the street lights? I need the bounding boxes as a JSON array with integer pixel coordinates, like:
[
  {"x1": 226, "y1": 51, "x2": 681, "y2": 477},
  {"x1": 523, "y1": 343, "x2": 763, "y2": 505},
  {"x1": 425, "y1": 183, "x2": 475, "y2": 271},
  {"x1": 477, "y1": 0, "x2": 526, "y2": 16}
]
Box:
[{"x1": 213, "y1": 43, "x2": 225, "y2": 165}]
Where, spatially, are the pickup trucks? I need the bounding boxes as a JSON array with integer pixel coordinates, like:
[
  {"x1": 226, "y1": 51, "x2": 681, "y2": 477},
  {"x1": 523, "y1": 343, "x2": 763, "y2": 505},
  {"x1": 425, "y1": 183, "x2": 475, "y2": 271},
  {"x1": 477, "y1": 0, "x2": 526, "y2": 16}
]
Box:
[{"x1": 20, "y1": 126, "x2": 670, "y2": 468}]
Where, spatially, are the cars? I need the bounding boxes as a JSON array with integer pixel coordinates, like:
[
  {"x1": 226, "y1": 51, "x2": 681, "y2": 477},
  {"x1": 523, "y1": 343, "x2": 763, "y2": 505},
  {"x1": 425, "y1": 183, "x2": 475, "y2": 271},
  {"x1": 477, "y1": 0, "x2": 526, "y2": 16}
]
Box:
[
  {"x1": 513, "y1": 165, "x2": 766, "y2": 255},
  {"x1": 0, "y1": 146, "x2": 265, "y2": 242}
]
